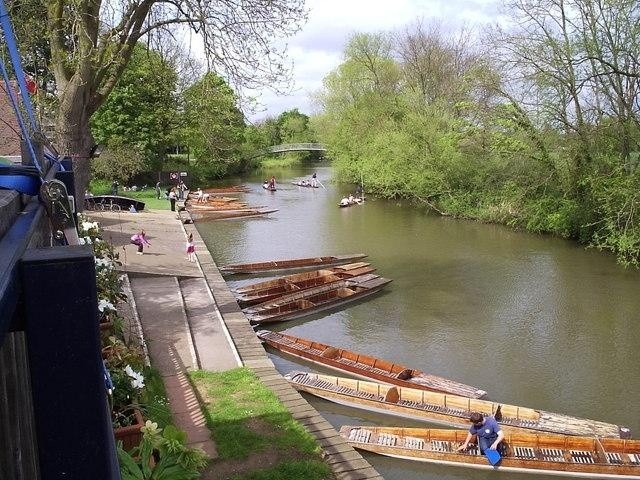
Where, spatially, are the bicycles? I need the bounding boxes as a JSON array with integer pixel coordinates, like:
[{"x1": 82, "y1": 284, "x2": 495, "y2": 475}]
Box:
[{"x1": 93, "y1": 197, "x2": 121, "y2": 213}]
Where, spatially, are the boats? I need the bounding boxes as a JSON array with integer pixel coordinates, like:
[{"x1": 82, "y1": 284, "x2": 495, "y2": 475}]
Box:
[
  {"x1": 254, "y1": 328, "x2": 489, "y2": 401},
  {"x1": 337, "y1": 424, "x2": 640, "y2": 479},
  {"x1": 84, "y1": 195, "x2": 146, "y2": 211},
  {"x1": 282, "y1": 369, "x2": 632, "y2": 443},
  {"x1": 291, "y1": 181, "x2": 319, "y2": 188},
  {"x1": 336, "y1": 196, "x2": 367, "y2": 207},
  {"x1": 188, "y1": 180, "x2": 280, "y2": 222},
  {"x1": 219, "y1": 250, "x2": 396, "y2": 327}
]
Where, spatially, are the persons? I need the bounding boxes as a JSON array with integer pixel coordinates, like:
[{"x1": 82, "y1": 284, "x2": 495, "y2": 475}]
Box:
[
  {"x1": 309, "y1": 172, "x2": 319, "y2": 188},
  {"x1": 263, "y1": 175, "x2": 276, "y2": 189},
  {"x1": 456, "y1": 411, "x2": 510, "y2": 457},
  {"x1": 185, "y1": 232, "x2": 196, "y2": 262},
  {"x1": 129, "y1": 228, "x2": 153, "y2": 256},
  {"x1": 84, "y1": 170, "x2": 209, "y2": 212},
  {"x1": 340, "y1": 184, "x2": 363, "y2": 207}
]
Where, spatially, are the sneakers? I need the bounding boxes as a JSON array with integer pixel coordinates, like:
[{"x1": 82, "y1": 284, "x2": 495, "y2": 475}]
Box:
[
  {"x1": 137, "y1": 252, "x2": 143, "y2": 255},
  {"x1": 188, "y1": 259, "x2": 195, "y2": 262}
]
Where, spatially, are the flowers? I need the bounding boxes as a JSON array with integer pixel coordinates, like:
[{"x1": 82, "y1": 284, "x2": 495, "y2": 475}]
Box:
[{"x1": 76, "y1": 211, "x2": 210, "y2": 480}]
